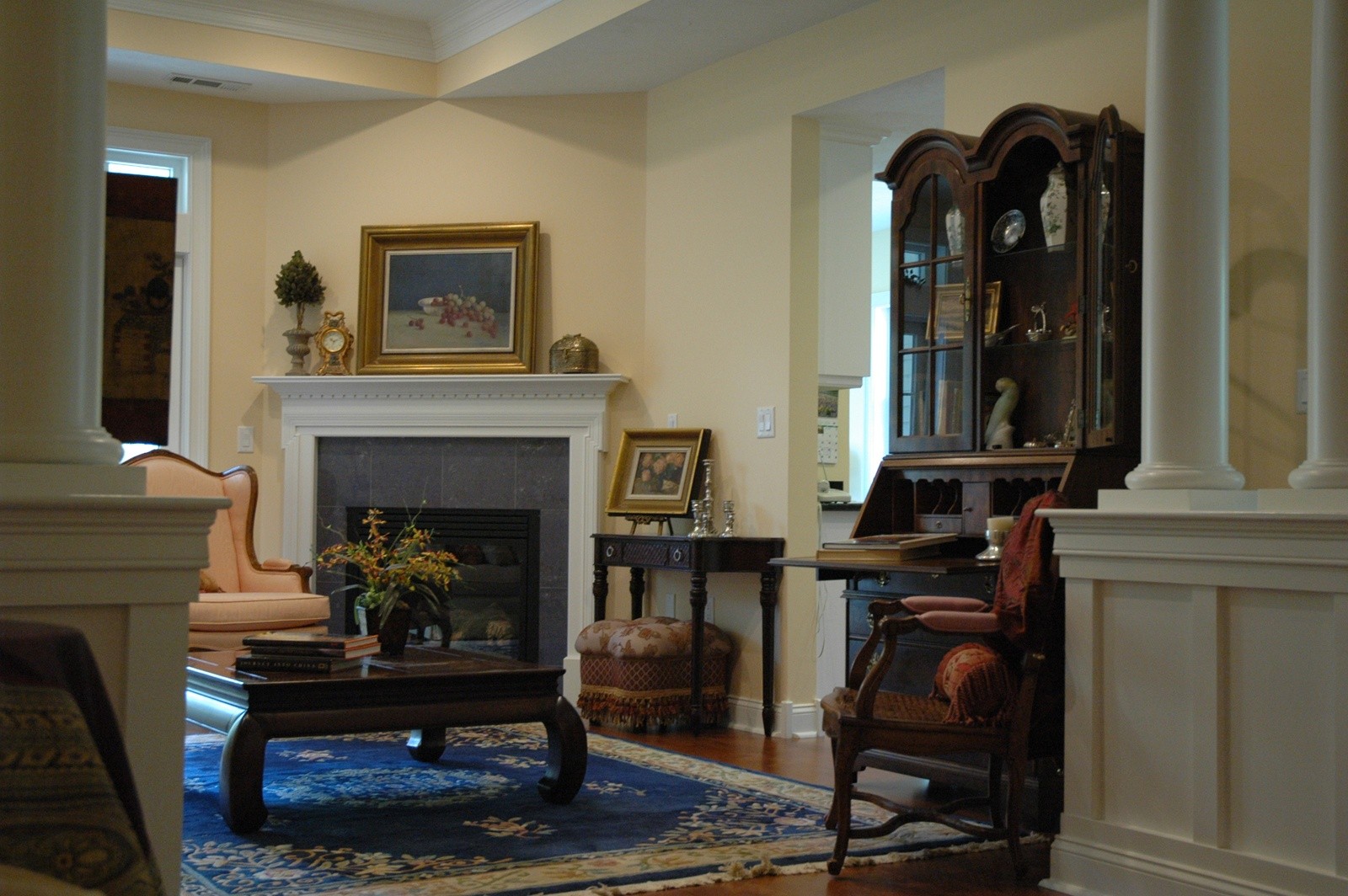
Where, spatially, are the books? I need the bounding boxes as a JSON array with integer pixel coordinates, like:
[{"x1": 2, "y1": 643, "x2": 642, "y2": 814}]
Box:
[{"x1": 236, "y1": 632, "x2": 380, "y2": 674}]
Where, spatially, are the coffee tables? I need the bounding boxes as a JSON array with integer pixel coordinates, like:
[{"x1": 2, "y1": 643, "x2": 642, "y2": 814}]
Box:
[{"x1": 183, "y1": 650, "x2": 586, "y2": 834}]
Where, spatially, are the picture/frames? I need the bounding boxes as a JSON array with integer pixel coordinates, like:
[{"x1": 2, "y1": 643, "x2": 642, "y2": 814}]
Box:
[
  {"x1": 359, "y1": 221, "x2": 538, "y2": 374},
  {"x1": 924, "y1": 280, "x2": 1001, "y2": 341},
  {"x1": 607, "y1": 427, "x2": 711, "y2": 517}
]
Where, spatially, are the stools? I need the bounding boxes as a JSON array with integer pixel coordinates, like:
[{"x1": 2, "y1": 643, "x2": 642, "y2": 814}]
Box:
[{"x1": 575, "y1": 614, "x2": 731, "y2": 733}]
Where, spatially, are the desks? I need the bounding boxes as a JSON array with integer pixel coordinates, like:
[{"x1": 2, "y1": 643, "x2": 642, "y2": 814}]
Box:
[{"x1": 591, "y1": 529, "x2": 785, "y2": 740}]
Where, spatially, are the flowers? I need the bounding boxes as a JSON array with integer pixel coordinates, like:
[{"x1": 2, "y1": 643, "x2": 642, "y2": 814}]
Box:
[{"x1": 313, "y1": 496, "x2": 460, "y2": 636}]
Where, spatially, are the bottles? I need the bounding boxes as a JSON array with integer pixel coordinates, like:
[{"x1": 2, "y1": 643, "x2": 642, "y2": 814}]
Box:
[
  {"x1": 1100, "y1": 173, "x2": 1111, "y2": 245},
  {"x1": 945, "y1": 204, "x2": 964, "y2": 264},
  {"x1": 1041, "y1": 162, "x2": 1068, "y2": 252}
]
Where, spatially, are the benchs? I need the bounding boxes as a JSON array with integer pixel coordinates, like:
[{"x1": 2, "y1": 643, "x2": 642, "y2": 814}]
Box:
[{"x1": 1, "y1": 616, "x2": 169, "y2": 896}]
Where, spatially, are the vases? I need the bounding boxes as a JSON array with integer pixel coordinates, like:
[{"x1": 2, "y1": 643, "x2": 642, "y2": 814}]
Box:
[
  {"x1": 370, "y1": 601, "x2": 413, "y2": 655},
  {"x1": 943, "y1": 199, "x2": 963, "y2": 263},
  {"x1": 1039, "y1": 166, "x2": 1066, "y2": 253}
]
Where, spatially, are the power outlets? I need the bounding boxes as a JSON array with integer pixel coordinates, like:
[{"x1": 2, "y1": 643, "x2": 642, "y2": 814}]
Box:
[
  {"x1": 664, "y1": 593, "x2": 675, "y2": 616},
  {"x1": 704, "y1": 596, "x2": 714, "y2": 623}
]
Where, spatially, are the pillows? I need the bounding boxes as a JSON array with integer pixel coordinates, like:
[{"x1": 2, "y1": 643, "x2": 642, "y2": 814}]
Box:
[{"x1": 933, "y1": 642, "x2": 1016, "y2": 724}]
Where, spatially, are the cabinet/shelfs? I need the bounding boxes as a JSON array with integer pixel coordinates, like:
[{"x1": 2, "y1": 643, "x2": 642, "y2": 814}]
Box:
[{"x1": 770, "y1": 100, "x2": 1143, "y2": 829}]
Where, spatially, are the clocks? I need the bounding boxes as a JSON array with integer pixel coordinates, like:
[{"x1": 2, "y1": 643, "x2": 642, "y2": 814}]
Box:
[{"x1": 315, "y1": 310, "x2": 353, "y2": 375}]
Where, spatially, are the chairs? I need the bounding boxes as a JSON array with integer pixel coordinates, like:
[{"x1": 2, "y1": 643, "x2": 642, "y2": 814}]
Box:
[
  {"x1": 825, "y1": 489, "x2": 1064, "y2": 878},
  {"x1": 120, "y1": 449, "x2": 332, "y2": 659}
]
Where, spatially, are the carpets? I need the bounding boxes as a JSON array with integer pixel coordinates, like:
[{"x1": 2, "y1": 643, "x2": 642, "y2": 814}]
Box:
[{"x1": 180, "y1": 721, "x2": 1045, "y2": 896}]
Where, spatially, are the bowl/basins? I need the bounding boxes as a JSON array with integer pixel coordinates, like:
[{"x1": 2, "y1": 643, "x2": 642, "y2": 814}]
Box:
[
  {"x1": 990, "y1": 209, "x2": 1027, "y2": 253},
  {"x1": 1026, "y1": 330, "x2": 1051, "y2": 342}
]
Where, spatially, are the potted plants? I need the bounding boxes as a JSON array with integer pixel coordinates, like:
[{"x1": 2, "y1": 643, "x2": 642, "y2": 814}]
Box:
[{"x1": 275, "y1": 249, "x2": 326, "y2": 374}]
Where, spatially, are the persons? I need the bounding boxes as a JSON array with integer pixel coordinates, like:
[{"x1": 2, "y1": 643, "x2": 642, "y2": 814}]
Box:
[{"x1": 915, "y1": 372, "x2": 963, "y2": 436}]
